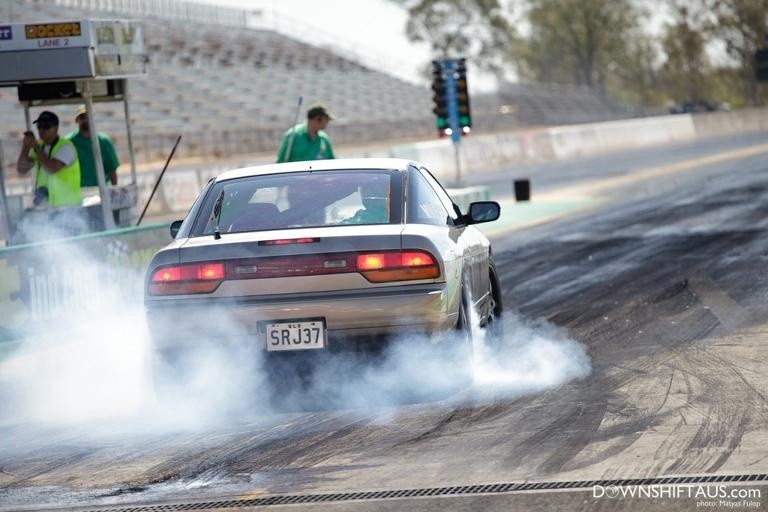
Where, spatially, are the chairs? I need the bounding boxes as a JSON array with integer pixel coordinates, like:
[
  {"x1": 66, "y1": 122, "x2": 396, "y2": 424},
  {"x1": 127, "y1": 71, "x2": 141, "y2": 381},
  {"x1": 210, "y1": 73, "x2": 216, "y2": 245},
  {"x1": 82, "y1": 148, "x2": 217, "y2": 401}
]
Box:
[{"x1": 229, "y1": 203, "x2": 287, "y2": 231}]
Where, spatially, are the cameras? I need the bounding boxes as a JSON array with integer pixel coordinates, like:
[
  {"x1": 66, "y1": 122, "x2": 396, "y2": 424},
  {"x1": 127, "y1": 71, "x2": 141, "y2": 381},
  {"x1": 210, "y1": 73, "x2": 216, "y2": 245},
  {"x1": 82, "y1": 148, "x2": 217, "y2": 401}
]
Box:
[{"x1": 33, "y1": 187, "x2": 47, "y2": 205}]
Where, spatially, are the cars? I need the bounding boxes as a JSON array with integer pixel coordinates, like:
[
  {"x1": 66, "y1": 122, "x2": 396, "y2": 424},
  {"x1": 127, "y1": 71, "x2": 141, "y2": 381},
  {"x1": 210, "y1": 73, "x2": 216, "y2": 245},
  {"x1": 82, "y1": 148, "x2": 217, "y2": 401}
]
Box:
[
  {"x1": 145, "y1": 157, "x2": 503, "y2": 382},
  {"x1": 671, "y1": 98, "x2": 729, "y2": 113}
]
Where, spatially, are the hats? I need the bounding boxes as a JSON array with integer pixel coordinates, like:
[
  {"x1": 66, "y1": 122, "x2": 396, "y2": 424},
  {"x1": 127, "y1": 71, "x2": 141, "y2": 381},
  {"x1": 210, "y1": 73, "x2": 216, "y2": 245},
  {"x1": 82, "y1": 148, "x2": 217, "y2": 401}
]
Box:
[
  {"x1": 75, "y1": 105, "x2": 87, "y2": 121},
  {"x1": 308, "y1": 107, "x2": 332, "y2": 120},
  {"x1": 33, "y1": 111, "x2": 58, "y2": 124}
]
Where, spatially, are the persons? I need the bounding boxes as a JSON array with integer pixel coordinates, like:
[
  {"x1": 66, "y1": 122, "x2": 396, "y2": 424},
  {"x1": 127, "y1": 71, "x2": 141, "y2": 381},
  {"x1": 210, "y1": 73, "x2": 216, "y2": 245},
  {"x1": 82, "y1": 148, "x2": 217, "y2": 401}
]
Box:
[
  {"x1": 276, "y1": 102, "x2": 336, "y2": 163},
  {"x1": 16, "y1": 104, "x2": 120, "y2": 206}
]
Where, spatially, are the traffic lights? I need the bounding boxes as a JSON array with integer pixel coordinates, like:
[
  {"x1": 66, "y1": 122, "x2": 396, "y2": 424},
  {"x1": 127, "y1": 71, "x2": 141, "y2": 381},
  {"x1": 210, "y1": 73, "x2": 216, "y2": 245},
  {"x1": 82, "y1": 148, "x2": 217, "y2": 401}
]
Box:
[{"x1": 430, "y1": 56, "x2": 472, "y2": 138}]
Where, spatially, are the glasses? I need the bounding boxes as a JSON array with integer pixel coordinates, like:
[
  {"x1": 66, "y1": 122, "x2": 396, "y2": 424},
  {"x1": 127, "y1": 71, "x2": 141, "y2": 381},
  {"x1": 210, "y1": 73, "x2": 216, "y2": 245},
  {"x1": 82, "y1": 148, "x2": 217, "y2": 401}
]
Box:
[{"x1": 38, "y1": 125, "x2": 51, "y2": 130}]
